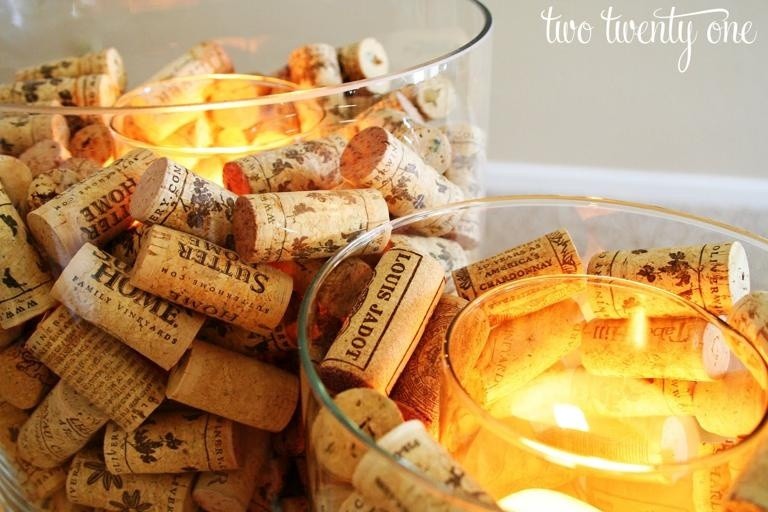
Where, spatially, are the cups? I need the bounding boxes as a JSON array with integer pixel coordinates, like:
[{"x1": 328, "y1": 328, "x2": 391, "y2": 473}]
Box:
[{"x1": 1, "y1": 0, "x2": 768, "y2": 512}]
